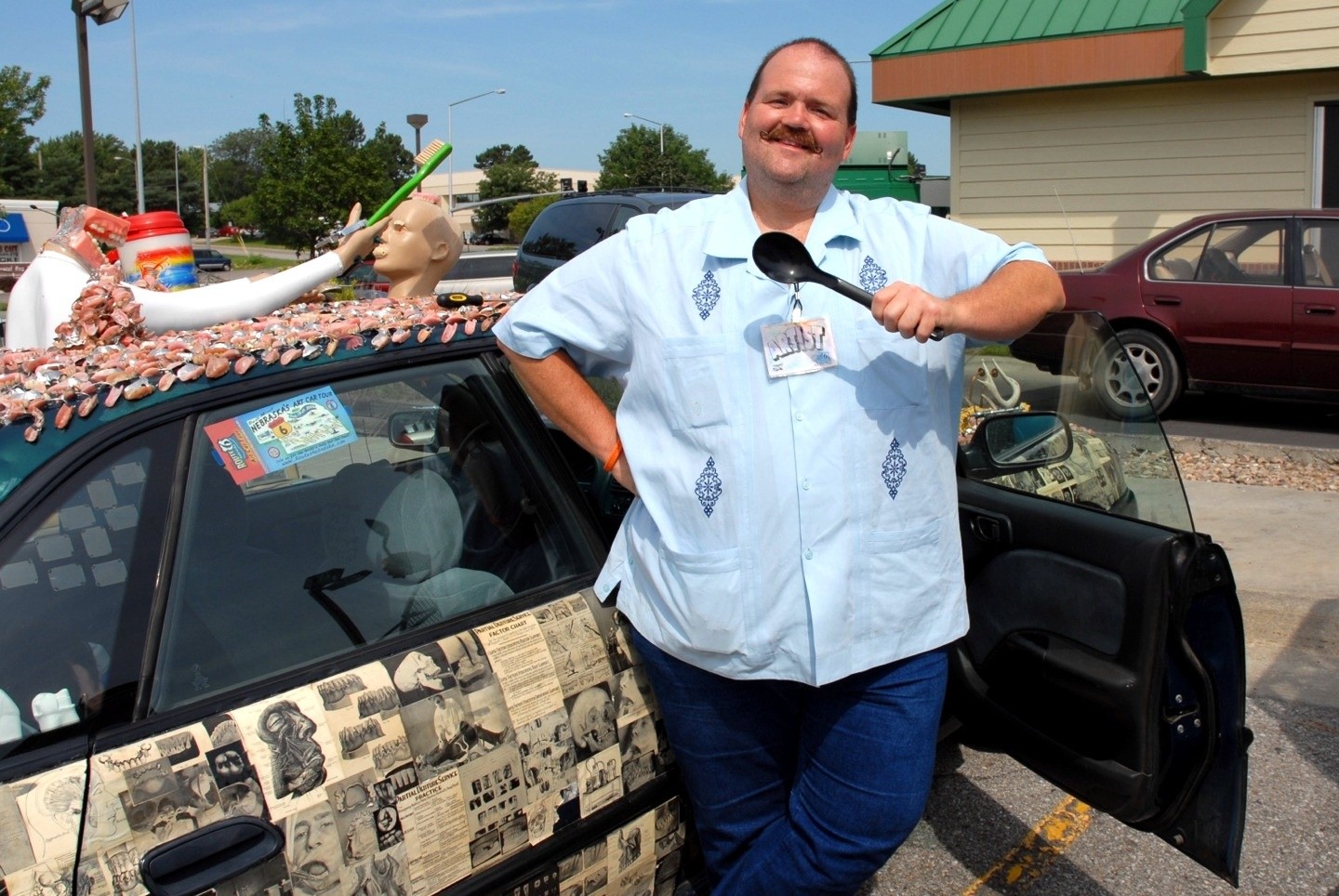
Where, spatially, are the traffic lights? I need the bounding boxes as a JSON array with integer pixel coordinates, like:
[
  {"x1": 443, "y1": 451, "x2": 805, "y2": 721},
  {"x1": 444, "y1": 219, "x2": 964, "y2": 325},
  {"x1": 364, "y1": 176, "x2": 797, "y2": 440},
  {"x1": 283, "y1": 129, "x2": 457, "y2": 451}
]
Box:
[
  {"x1": 560, "y1": 178, "x2": 573, "y2": 200},
  {"x1": 577, "y1": 180, "x2": 588, "y2": 193}
]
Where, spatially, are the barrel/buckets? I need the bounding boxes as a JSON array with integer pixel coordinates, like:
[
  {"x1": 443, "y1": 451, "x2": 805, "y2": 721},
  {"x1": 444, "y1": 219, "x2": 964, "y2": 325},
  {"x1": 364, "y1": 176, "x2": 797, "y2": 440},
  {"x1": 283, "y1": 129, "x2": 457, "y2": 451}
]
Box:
[{"x1": 116, "y1": 210, "x2": 199, "y2": 293}]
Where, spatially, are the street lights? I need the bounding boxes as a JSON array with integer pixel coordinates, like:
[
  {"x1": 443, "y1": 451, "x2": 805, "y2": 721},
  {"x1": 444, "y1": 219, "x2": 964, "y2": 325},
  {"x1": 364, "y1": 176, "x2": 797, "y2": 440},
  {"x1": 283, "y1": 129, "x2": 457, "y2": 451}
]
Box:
[
  {"x1": 448, "y1": 88, "x2": 505, "y2": 217},
  {"x1": 114, "y1": 156, "x2": 138, "y2": 204},
  {"x1": 175, "y1": 145, "x2": 202, "y2": 216},
  {"x1": 405, "y1": 113, "x2": 429, "y2": 193},
  {"x1": 69, "y1": 0, "x2": 129, "y2": 208},
  {"x1": 624, "y1": 113, "x2": 665, "y2": 192}
]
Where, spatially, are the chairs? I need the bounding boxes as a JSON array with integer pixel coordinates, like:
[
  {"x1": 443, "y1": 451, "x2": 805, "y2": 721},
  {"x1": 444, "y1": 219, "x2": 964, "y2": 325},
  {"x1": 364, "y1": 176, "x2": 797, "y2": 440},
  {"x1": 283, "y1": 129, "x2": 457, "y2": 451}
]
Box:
[
  {"x1": 172, "y1": 467, "x2": 316, "y2": 655},
  {"x1": 300, "y1": 464, "x2": 517, "y2": 652},
  {"x1": 1302, "y1": 243, "x2": 1336, "y2": 287}
]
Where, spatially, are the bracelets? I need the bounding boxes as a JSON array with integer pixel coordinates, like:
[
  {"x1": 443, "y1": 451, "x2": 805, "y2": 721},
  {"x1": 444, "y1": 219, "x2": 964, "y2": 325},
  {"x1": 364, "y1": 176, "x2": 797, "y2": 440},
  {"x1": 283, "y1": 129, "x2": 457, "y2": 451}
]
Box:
[{"x1": 604, "y1": 439, "x2": 622, "y2": 471}]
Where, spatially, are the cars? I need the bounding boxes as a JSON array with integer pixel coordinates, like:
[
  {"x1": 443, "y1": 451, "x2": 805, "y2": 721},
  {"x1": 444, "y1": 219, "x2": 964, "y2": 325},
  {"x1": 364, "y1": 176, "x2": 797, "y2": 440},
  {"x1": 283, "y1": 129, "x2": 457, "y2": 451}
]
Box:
[
  {"x1": 192, "y1": 248, "x2": 232, "y2": 272},
  {"x1": 0, "y1": 293, "x2": 1255, "y2": 896},
  {"x1": 320, "y1": 249, "x2": 519, "y2": 301},
  {"x1": 197, "y1": 224, "x2": 240, "y2": 238},
  {"x1": 473, "y1": 232, "x2": 509, "y2": 246},
  {"x1": 1009, "y1": 209, "x2": 1339, "y2": 424}
]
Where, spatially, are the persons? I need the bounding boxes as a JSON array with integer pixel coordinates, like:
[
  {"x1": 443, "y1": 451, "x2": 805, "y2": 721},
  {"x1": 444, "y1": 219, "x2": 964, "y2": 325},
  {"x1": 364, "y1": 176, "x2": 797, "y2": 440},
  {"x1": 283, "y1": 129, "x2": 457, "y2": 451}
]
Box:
[
  {"x1": 374, "y1": 198, "x2": 462, "y2": 300},
  {"x1": 488, "y1": 36, "x2": 1066, "y2": 896},
  {"x1": 4, "y1": 203, "x2": 393, "y2": 353}
]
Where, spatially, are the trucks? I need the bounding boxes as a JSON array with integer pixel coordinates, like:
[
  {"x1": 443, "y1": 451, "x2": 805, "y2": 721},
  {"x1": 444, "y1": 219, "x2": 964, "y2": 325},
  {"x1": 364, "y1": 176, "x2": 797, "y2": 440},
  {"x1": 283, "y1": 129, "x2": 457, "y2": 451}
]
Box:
[{"x1": 0, "y1": 198, "x2": 60, "y2": 279}]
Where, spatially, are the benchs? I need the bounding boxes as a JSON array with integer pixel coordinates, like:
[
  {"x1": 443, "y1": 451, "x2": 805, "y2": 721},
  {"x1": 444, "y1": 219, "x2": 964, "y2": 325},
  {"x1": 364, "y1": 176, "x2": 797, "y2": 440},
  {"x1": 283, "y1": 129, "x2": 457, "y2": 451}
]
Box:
[{"x1": 1151, "y1": 248, "x2": 1252, "y2": 285}]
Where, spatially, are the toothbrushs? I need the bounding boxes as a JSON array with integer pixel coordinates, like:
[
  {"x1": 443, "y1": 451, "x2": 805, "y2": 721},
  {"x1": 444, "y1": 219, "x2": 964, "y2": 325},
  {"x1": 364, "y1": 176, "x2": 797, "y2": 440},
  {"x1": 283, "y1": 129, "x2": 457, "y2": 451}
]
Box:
[{"x1": 364, "y1": 139, "x2": 455, "y2": 227}]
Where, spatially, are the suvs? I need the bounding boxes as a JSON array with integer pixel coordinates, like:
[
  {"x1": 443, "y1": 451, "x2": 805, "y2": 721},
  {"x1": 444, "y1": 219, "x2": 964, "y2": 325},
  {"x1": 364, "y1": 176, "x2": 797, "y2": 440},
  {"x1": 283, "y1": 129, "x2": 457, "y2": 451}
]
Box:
[{"x1": 511, "y1": 187, "x2": 718, "y2": 293}]
